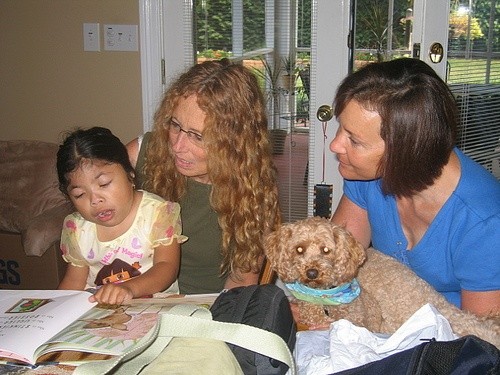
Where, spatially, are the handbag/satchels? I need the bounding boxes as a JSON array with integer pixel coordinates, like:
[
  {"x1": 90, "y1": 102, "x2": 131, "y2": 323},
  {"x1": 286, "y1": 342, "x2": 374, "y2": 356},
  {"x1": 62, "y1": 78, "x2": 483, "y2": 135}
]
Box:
[
  {"x1": 209, "y1": 283, "x2": 297, "y2": 375},
  {"x1": 70, "y1": 304, "x2": 297, "y2": 375},
  {"x1": 327, "y1": 334, "x2": 500, "y2": 375}
]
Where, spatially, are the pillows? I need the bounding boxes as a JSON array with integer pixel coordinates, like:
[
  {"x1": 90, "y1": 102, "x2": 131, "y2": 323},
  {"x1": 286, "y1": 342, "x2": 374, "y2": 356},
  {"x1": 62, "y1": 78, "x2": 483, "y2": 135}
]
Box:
[{"x1": 0, "y1": 138, "x2": 77, "y2": 257}]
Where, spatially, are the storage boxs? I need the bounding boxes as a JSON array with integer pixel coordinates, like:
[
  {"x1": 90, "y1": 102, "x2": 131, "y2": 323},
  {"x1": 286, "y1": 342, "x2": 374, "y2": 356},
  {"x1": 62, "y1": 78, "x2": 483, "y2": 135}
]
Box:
[{"x1": 0, "y1": 233, "x2": 64, "y2": 290}]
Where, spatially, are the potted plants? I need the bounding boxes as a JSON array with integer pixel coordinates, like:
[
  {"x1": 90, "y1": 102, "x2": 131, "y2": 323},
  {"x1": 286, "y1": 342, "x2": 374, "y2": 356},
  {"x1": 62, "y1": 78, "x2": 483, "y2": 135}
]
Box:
[{"x1": 249, "y1": 53, "x2": 300, "y2": 155}]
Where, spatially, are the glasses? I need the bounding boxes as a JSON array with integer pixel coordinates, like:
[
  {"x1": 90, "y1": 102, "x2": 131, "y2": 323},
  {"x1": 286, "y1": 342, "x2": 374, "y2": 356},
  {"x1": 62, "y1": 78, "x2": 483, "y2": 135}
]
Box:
[{"x1": 164, "y1": 119, "x2": 203, "y2": 148}]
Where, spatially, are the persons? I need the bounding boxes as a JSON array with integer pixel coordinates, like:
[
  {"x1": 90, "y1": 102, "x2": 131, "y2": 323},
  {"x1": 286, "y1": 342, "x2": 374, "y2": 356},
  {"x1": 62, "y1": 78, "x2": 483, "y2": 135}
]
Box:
[
  {"x1": 125, "y1": 57, "x2": 279, "y2": 295},
  {"x1": 56, "y1": 128, "x2": 188, "y2": 305},
  {"x1": 290, "y1": 58, "x2": 500, "y2": 327}
]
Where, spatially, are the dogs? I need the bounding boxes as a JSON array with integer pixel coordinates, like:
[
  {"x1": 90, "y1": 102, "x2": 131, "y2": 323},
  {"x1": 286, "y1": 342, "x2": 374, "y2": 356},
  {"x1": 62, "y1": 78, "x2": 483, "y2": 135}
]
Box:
[{"x1": 260, "y1": 214, "x2": 500, "y2": 350}]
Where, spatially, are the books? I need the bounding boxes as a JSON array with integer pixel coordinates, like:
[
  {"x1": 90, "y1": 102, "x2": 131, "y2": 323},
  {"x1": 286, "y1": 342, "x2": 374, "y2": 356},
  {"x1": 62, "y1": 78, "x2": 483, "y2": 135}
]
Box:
[{"x1": 0, "y1": 295, "x2": 159, "y2": 365}]
